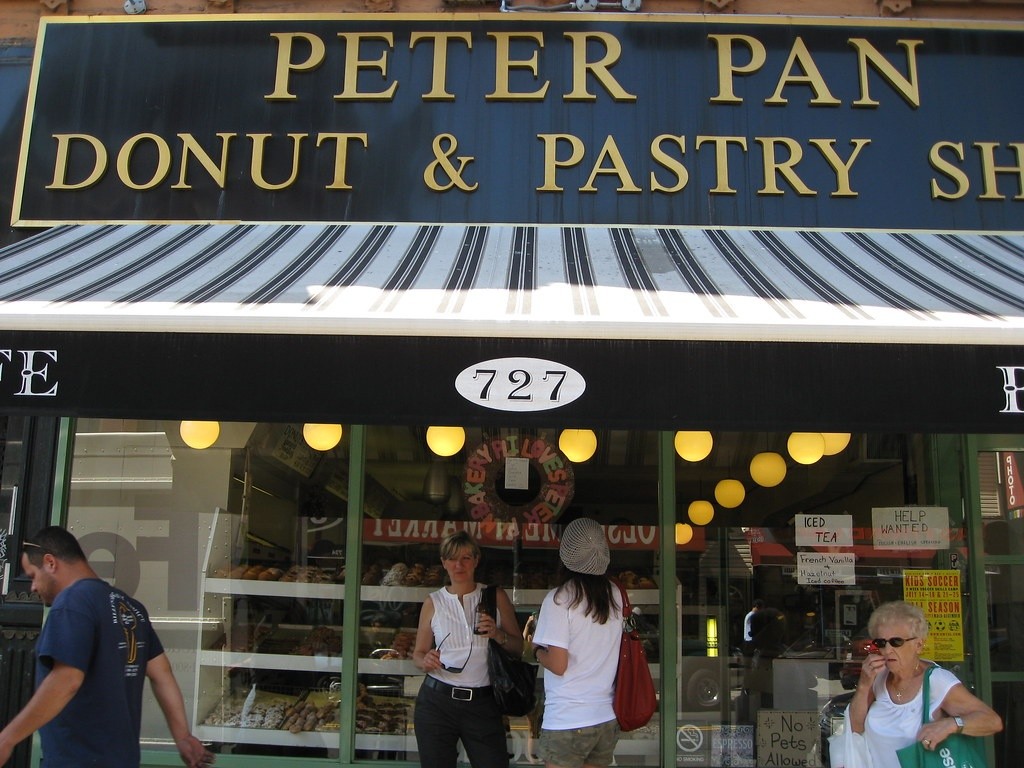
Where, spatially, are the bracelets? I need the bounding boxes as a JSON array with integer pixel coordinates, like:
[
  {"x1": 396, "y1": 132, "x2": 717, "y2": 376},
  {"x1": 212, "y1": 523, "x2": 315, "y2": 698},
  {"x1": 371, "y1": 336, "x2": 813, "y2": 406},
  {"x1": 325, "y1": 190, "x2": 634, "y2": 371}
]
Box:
[
  {"x1": 533, "y1": 645, "x2": 548, "y2": 663},
  {"x1": 499, "y1": 632, "x2": 508, "y2": 644}
]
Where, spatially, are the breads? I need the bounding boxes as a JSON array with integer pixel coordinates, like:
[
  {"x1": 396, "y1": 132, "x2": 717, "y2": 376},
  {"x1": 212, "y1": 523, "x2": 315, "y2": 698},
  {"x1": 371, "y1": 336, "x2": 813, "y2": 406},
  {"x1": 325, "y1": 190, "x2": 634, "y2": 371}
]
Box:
[
  {"x1": 503, "y1": 699, "x2": 543, "y2": 739},
  {"x1": 481, "y1": 566, "x2": 654, "y2": 590},
  {"x1": 202, "y1": 562, "x2": 449, "y2": 735},
  {"x1": 633, "y1": 712, "x2": 658, "y2": 734}
]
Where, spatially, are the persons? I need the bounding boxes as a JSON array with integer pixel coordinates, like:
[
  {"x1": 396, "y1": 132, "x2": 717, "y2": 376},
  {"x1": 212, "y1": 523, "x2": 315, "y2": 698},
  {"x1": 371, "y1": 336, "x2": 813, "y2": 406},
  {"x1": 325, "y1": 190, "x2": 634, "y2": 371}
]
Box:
[
  {"x1": 413, "y1": 530, "x2": 524, "y2": 768},
  {"x1": 0, "y1": 525, "x2": 218, "y2": 768},
  {"x1": 528, "y1": 518, "x2": 628, "y2": 768},
  {"x1": 849, "y1": 601, "x2": 1003, "y2": 768},
  {"x1": 283, "y1": 540, "x2": 338, "y2": 690}
]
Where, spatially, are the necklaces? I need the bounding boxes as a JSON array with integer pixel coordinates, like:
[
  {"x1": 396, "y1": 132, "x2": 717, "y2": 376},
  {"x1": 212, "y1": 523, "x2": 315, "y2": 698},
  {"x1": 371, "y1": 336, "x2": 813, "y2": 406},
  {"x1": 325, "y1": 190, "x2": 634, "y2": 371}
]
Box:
[{"x1": 896, "y1": 663, "x2": 918, "y2": 700}]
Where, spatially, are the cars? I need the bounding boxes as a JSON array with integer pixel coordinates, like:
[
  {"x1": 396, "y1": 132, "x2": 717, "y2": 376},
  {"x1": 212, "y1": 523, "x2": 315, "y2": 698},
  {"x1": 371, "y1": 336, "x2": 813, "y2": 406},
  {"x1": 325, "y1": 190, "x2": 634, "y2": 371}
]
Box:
[{"x1": 644, "y1": 636, "x2": 747, "y2": 713}]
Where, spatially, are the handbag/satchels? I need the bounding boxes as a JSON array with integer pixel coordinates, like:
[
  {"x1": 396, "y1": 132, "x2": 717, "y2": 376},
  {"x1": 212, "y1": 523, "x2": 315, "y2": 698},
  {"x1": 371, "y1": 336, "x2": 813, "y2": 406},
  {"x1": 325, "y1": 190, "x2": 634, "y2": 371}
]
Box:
[
  {"x1": 609, "y1": 575, "x2": 657, "y2": 732},
  {"x1": 896, "y1": 666, "x2": 986, "y2": 768},
  {"x1": 484, "y1": 583, "x2": 539, "y2": 717},
  {"x1": 734, "y1": 687, "x2": 756, "y2": 759},
  {"x1": 827, "y1": 702, "x2": 875, "y2": 768}
]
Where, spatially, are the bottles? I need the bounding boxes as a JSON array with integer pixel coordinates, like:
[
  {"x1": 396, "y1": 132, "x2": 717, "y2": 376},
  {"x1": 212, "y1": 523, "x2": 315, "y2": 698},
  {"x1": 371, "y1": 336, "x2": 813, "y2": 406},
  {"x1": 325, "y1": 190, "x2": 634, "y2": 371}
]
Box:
[{"x1": 473, "y1": 588, "x2": 489, "y2": 635}]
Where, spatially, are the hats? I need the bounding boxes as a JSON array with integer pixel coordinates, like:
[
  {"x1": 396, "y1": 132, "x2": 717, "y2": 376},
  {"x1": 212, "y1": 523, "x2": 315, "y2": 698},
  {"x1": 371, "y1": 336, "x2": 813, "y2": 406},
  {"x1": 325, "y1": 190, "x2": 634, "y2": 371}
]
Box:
[{"x1": 559, "y1": 518, "x2": 610, "y2": 576}]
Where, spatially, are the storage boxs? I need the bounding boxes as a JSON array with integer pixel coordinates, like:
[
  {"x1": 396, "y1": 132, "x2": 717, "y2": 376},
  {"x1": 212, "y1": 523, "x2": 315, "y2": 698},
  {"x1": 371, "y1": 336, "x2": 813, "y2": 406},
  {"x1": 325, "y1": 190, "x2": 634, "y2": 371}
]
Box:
[
  {"x1": 756, "y1": 658, "x2": 830, "y2": 767},
  {"x1": 676, "y1": 720, "x2": 754, "y2": 768}
]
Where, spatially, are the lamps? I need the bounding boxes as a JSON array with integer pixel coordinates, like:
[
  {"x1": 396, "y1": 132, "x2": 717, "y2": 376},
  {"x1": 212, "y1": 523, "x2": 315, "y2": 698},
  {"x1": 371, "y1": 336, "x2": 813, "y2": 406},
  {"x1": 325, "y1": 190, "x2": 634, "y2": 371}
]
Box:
[
  {"x1": 714, "y1": 456, "x2": 745, "y2": 508},
  {"x1": 558, "y1": 429, "x2": 597, "y2": 463},
  {"x1": 674, "y1": 431, "x2": 713, "y2": 462},
  {"x1": 688, "y1": 480, "x2": 714, "y2": 526},
  {"x1": 302, "y1": 423, "x2": 343, "y2": 451},
  {"x1": 426, "y1": 426, "x2": 465, "y2": 457},
  {"x1": 179, "y1": 421, "x2": 220, "y2": 450},
  {"x1": 788, "y1": 433, "x2": 851, "y2": 465},
  {"x1": 675, "y1": 504, "x2": 693, "y2": 544},
  {"x1": 749, "y1": 432, "x2": 787, "y2": 487}
]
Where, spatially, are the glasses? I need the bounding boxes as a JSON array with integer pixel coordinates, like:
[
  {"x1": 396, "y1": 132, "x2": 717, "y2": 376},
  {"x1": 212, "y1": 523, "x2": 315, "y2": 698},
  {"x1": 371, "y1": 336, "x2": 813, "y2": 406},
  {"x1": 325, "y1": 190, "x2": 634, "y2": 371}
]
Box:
[
  {"x1": 871, "y1": 637, "x2": 917, "y2": 648},
  {"x1": 435, "y1": 633, "x2": 473, "y2": 673},
  {"x1": 23, "y1": 539, "x2": 53, "y2": 554},
  {"x1": 748, "y1": 632, "x2": 753, "y2": 637}
]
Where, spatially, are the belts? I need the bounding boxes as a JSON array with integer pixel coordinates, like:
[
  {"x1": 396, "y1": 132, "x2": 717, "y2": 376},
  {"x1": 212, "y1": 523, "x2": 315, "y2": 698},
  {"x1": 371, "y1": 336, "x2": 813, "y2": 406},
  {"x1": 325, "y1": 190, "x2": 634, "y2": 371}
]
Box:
[{"x1": 423, "y1": 675, "x2": 493, "y2": 701}]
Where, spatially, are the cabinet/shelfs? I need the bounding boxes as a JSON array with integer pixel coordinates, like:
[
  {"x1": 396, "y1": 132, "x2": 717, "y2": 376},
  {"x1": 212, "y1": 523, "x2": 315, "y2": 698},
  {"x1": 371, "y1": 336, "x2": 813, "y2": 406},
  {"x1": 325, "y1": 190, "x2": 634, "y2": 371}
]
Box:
[{"x1": 192, "y1": 507, "x2": 683, "y2": 767}]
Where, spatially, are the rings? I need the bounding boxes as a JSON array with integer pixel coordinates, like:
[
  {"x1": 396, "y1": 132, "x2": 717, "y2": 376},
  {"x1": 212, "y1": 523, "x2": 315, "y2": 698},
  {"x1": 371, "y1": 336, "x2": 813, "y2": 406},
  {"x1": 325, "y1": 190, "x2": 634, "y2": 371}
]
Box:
[{"x1": 924, "y1": 740, "x2": 930, "y2": 744}]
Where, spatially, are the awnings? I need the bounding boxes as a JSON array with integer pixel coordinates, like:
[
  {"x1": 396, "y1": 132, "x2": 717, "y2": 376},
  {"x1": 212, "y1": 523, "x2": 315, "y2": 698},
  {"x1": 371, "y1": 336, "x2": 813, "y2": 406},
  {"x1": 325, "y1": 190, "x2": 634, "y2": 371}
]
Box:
[{"x1": 0, "y1": 214, "x2": 1024, "y2": 435}]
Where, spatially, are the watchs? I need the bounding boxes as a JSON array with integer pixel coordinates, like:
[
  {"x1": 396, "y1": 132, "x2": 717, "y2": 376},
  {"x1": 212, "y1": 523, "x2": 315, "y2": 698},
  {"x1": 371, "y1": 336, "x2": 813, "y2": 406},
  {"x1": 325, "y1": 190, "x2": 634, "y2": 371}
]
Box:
[{"x1": 953, "y1": 716, "x2": 964, "y2": 734}]
